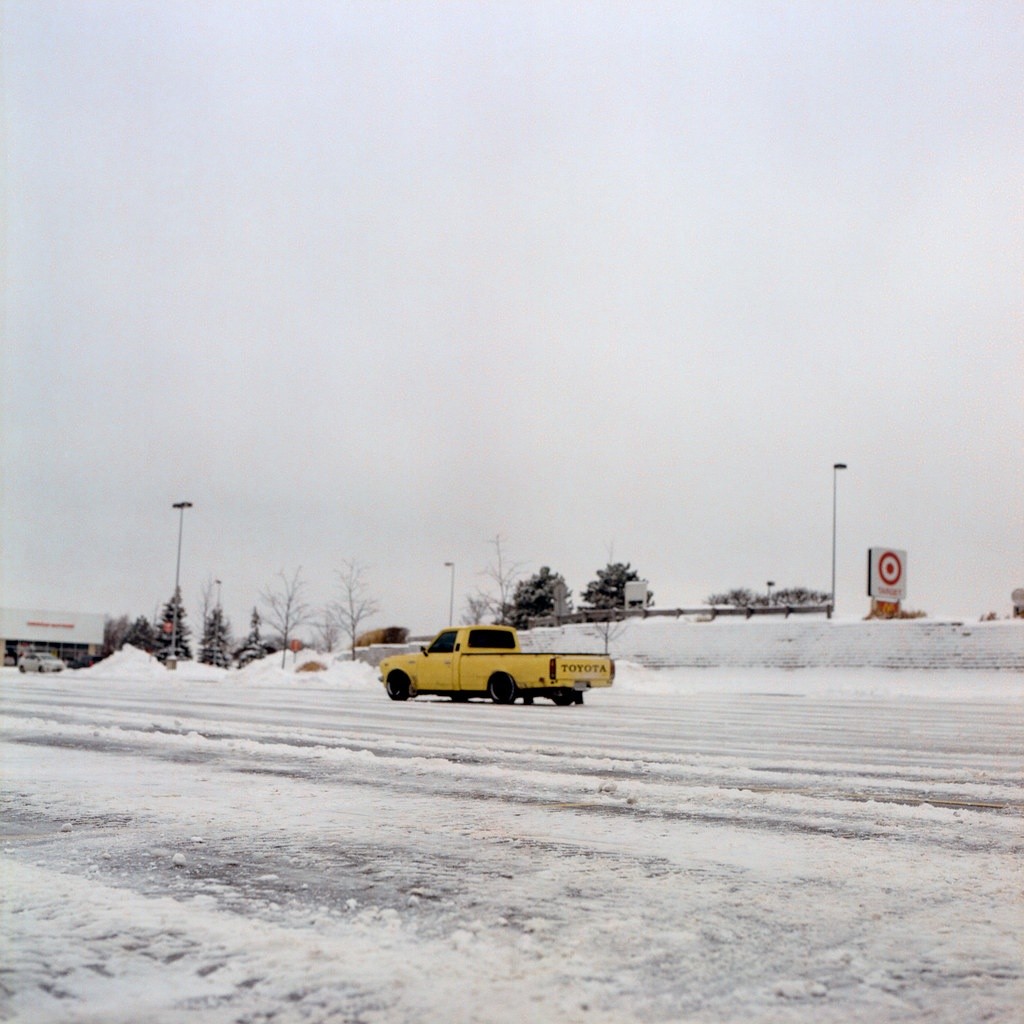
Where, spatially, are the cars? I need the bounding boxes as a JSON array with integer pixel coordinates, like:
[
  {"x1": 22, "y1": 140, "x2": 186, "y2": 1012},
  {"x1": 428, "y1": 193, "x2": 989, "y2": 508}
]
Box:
[
  {"x1": 64, "y1": 655, "x2": 102, "y2": 669},
  {"x1": 17, "y1": 653, "x2": 63, "y2": 673}
]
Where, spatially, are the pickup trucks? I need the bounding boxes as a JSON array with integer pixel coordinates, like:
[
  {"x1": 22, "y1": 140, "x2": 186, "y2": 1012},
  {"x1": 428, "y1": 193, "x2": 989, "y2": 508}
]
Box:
[{"x1": 380, "y1": 624, "x2": 615, "y2": 706}]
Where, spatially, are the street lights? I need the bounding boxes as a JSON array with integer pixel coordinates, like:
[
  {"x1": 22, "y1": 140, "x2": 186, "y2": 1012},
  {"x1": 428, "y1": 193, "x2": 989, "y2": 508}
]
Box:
[
  {"x1": 830, "y1": 464, "x2": 847, "y2": 610},
  {"x1": 445, "y1": 562, "x2": 454, "y2": 626},
  {"x1": 170, "y1": 502, "x2": 192, "y2": 657}
]
